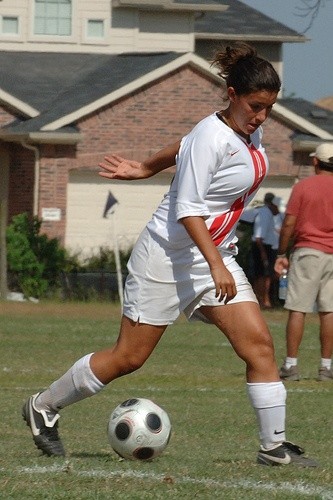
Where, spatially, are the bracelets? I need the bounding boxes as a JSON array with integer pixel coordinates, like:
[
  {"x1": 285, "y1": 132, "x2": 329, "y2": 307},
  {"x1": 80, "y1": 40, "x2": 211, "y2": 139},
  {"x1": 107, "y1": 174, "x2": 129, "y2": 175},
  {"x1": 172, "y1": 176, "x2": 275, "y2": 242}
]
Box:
[{"x1": 277, "y1": 250, "x2": 286, "y2": 257}]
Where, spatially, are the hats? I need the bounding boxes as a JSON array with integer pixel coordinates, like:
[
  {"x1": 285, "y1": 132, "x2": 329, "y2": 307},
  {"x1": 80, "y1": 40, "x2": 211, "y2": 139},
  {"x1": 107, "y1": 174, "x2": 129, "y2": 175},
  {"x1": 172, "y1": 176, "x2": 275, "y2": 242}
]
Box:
[
  {"x1": 272, "y1": 197, "x2": 286, "y2": 212},
  {"x1": 309, "y1": 144, "x2": 333, "y2": 164}
]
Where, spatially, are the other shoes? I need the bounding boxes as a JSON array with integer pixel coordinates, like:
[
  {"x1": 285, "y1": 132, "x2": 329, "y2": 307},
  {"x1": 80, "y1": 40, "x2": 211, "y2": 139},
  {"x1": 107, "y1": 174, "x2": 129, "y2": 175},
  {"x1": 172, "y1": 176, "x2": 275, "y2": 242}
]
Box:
[{"x1": 271, "y1": 299, "x2": 285, "y2": 308}]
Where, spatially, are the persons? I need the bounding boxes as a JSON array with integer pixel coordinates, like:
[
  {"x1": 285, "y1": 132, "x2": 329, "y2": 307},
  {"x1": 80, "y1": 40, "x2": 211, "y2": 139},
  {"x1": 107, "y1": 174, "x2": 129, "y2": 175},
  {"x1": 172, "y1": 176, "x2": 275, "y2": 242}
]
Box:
[
  {"x1": 264, "y1": 192, "x2": 284, "y2": 310},
  {"x1": 252, "y1": 196, "x2": 285, "y2": 309},
  {"x1": 21, "y1": 41, "x2": 317, "y2": 468},
  {"x1": 273, "y1": 142, "x2": 333, "y2": 381}
]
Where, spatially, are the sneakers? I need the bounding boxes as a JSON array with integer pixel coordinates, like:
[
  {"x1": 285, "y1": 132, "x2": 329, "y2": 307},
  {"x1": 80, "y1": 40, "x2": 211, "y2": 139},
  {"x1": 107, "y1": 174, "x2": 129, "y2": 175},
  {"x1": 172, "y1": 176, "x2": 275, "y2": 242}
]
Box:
[
  {"x1": 22, "y1": 391, "x2": 65, "y2": 459},
  {"x1": 318, "y1": 367, "x2": 333, "y2": 380},
  {"x1": 279, "y1": 358, "x2": 298, "y2": 381},
  {"x1": 256, "y1": 441, "x2": 318, "y2": 468}
]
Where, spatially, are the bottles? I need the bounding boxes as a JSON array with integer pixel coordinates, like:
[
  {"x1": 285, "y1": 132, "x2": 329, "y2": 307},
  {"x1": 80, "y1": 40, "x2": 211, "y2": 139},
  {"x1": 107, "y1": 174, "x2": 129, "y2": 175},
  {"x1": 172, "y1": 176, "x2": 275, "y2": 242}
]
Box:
[{"x1": 279, "y1": 270, "x2": 288, "y2": 299}]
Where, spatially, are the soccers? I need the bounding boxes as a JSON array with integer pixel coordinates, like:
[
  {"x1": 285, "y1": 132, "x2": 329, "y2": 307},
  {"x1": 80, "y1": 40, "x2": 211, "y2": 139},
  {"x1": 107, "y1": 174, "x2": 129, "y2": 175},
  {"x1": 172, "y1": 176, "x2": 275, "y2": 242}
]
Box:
[{"x1": 107, "y1": 397, "x2": 172, "y2": 461}]
